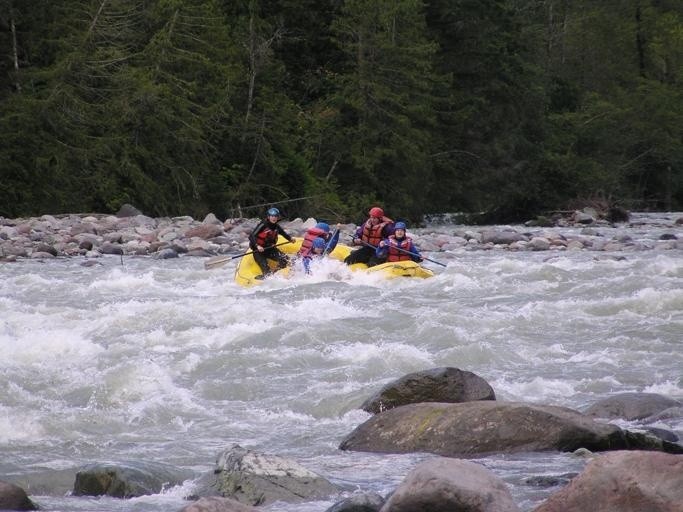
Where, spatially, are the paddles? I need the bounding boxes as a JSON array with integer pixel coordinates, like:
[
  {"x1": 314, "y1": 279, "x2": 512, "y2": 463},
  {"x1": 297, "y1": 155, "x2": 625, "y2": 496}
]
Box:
[
  {"x1": 342, "y1": 230, "x2": 377, "y2": 249},
  {"x1": 204, "y1": 241, "x2": 293, "y2": 270}
]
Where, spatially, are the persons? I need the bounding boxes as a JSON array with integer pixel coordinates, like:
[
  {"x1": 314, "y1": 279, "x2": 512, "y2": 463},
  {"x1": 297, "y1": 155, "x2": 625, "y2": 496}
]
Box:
[
  {"x1": 303, "y1": 222, "x2": 342, "y2": 277},
  {"x1": 297, "y1": 223, "x2": 332, "y2": 257},
  {"x1": 376, "y1": 221, "x2": 428, "y2": 263},
  {"x1": 344, "y1": 207, "x2": 395, "y2": 268},
  {"x1": 249, "y1": 208, "x2": 297, "y2": 280}
]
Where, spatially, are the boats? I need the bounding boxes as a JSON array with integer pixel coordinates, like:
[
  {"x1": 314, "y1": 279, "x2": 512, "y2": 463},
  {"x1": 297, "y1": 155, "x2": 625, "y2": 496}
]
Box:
[{"x1": 234, "y1": 233, "x2": 435, "y2": 287}]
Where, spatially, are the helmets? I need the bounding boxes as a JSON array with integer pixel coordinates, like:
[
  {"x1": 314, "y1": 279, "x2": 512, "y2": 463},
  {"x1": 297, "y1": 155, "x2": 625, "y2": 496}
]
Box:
[
  {"x1": 313, "y1": 238, "x2": 325, "y2": 248},
  {"x1": 266, "y1": 208, "x2": 279, "y2": 216},
  {"x1": 369, "y1": 208, "x2": 384, "y2": 218},
  {"x1": 394, "y1": 222, "x2": 406, "y2": 232},
  {"x1": 316, "y1": 223, "x2": 330, "y2": 232}
]
